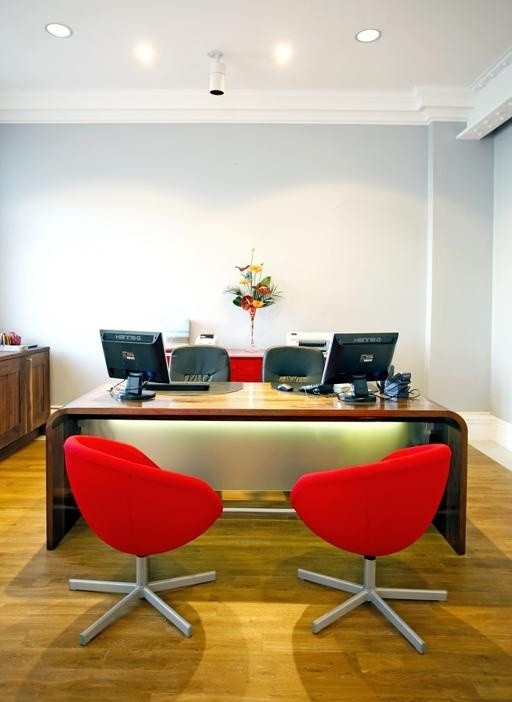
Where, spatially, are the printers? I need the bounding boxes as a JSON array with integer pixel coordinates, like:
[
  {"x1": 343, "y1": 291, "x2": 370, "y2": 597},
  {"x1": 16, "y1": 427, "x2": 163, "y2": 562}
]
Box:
[{"x1": 286, "y1": 331, "x2": 331, "y2": 358}]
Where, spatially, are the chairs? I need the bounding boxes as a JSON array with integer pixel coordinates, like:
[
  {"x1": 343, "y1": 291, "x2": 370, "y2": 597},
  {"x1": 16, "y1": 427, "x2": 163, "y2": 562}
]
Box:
[
  {"x1": 288, "y1": 442, "x2": 452, "y2": 655},
  {"x1": 169, "y1": 346, "x2": 230, "y2": 383},
  {"x1": 62, "y1": 434, "x2": 225, "y2": 647},
  {"x1": 263, "y1": 346, "x2": 325, "y2": 384}
]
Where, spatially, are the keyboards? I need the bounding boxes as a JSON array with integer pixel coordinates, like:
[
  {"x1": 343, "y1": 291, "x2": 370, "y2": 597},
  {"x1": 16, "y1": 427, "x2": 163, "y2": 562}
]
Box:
[
  {"x1": 143, "y1": 381, "x2": 209, "y2": 391},
  {"x1": 298, "y1": 383, "x2": 335, "y2": 394}
]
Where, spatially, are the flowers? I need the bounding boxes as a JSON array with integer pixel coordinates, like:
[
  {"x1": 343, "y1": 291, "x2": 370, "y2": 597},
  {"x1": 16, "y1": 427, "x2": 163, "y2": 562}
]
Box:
[{"x1": 223, "y1": 247, "x2": 288, "y2": 345}]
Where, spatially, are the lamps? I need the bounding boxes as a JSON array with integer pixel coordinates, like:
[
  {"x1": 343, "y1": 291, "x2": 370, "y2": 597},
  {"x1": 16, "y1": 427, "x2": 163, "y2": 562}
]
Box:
[{"x1": 208, "y1": 49, "x2": 225, "y2": 96}]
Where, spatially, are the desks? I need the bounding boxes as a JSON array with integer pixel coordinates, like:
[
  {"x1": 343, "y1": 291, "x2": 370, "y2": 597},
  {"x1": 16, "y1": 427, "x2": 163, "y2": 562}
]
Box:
[
  {"x1": 46, "y1": 378, "x2": 468, "y2": 555},
  {"x1": 164, "y1": 347, "x2": 264, "y2": 383}
]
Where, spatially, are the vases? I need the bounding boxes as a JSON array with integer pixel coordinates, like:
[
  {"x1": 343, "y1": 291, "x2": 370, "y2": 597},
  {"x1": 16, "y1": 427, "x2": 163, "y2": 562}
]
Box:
[{"x1": 244, "y1": 315, "x2": 260, "y2": 353}]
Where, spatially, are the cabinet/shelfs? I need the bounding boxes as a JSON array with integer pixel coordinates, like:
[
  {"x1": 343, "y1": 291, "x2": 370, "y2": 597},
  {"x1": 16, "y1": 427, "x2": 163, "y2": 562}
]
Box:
[{"x1": 0, "y1": 345, "x2": 50, "y2": 460}]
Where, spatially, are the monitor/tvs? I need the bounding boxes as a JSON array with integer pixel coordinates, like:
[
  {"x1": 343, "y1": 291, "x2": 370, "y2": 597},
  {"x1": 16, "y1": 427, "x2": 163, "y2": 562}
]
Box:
[
  {"x1": 321, "y1": 332, "x2": 399, "y2": 402},
  {"x1": 100, "y1": 329, "x2": 169, "y2": 401}
]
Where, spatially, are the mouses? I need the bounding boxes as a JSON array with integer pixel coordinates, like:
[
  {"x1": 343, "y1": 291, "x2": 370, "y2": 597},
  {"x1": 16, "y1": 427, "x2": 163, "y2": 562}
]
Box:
[{"x1": 277, "y1": 383, "x2": 293, "y2": 392}]
